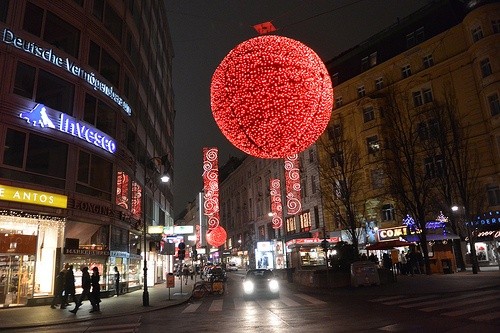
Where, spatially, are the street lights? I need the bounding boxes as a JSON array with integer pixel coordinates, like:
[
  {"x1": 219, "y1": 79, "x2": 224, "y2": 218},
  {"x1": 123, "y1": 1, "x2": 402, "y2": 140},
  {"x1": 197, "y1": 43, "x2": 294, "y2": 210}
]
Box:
[{"x1": 143, "y1": 157, "x2": 171, "y2": 308}]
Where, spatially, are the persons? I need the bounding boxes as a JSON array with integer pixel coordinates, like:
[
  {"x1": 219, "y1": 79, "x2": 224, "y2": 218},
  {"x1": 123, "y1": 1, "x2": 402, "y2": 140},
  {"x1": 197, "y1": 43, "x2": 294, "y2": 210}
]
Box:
[
  {"x1": 381, "y1": 253, "x2": 392, "y2": 270},
  {"x1": 113, "y1": 266, "x2": 120, "y2": 296},
  {"x1": 362, "y1": 253, "x2": 379, "y2": 265},
  {"x1": 50, "y1": 263, "x2": 82, "y2": 309},
  {"x1": 70, "y1": 267, "x2": 97, "y2": 314},
  {"x1": 90, "y1": 267, "x2": 101, "y2": 311},
  {"x1": 398, "y1": 250, "x2": 424, "y2": 274},
  {"x1": 178, "y1": 264, "x2": 194, "y2": 285}
]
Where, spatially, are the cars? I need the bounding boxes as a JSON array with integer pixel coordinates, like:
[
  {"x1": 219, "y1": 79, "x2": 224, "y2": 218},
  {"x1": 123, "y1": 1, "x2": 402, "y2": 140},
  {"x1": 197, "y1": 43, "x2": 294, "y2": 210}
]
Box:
[
  {"x1": 241, "y1": 269, "x2": 280, "y2": 301},
  {"x1": 174, "y1": 260, "x2": 239, "y2": 300}
]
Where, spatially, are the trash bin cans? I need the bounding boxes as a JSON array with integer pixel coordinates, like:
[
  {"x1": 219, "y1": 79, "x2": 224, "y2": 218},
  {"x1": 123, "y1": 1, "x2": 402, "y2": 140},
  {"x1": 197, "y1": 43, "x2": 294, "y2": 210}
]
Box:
[
  {"x1": 440, "y1": 258, "x2": 452, "y2": 274},
  {"x1": 284, "y1": 268, "x2": 296, "y2": 282}
]
